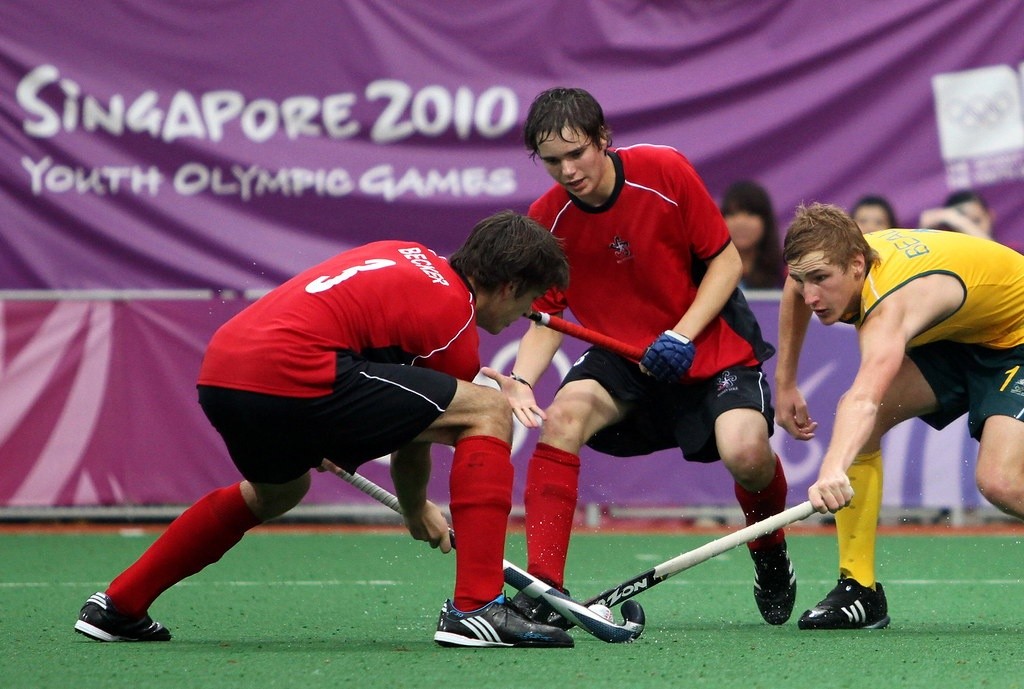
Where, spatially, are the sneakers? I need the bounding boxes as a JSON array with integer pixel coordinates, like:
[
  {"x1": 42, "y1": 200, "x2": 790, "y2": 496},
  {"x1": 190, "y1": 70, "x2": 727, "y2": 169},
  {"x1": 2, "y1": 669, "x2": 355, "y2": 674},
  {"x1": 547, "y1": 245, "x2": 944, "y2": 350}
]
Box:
[
  {"x1": 433, "y1": 590, "x2": 575, "y2": 650},
  {"x1": 73, "y1": 591, "x2": 171, "y2": 643},
  {"x1": 749, "y1": 539, "x2": 798, "y2": 625},
  {"x1": 509, "y1": 590, "x2": 576, "y2": 631},
  {"x1": 797, "y1": 577, "x2": 890, "y2": 629}
]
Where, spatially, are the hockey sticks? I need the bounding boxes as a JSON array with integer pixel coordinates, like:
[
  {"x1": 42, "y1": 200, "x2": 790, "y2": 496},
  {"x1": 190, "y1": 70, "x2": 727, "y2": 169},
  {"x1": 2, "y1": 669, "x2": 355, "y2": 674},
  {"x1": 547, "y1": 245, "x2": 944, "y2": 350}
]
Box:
[
  {"x1": 315, "y1": 458, "x2": 646, "y2": 643},
  {"x1": 524, "y1": 308, "x2": 645, "y2": 361},
  {"x1": 509, "y1": 501, "x2": 819, "y2": 631}
]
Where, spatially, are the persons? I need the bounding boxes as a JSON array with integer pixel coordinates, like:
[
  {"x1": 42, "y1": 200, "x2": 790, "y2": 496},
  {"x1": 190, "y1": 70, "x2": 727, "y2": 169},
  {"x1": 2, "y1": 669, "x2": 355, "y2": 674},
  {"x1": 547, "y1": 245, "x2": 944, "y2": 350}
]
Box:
[
  {"x1": 721, "y1": 177, "x2": 998, "y2": 289},
  {"x1": 74, "y1": 212, "x2": 570, "y2": 648},
  {"x1": 774, "y1": 200, "x2": 1024, "y2": 631},
  {"x1": 480, "y1": 87, "x2": 797, "y2": 631}
]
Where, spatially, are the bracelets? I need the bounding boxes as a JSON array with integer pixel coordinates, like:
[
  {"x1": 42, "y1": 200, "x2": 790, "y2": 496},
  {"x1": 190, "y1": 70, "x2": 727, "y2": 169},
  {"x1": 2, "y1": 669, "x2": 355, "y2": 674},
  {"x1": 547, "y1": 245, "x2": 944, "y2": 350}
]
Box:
[{"x1": 509, "y1": 371, "x2": 533, "y2": 389}]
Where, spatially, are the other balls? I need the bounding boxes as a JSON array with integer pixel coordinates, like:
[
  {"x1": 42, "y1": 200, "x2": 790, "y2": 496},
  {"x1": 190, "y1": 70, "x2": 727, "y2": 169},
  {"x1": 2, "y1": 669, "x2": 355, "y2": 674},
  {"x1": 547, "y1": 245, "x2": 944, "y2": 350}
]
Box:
[{"x1": 587, "y1": 604, "x2": 615, "y2": 625}]
